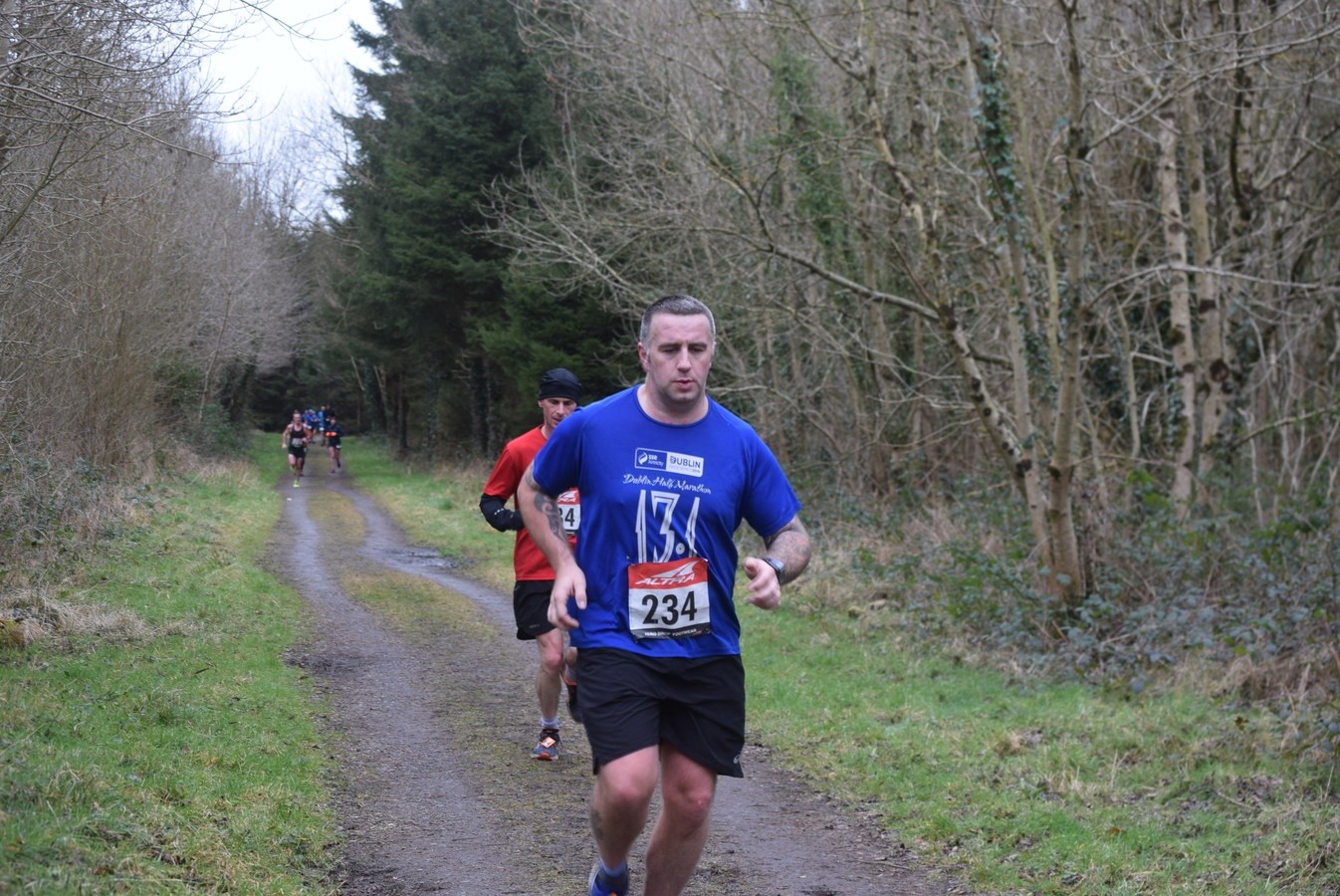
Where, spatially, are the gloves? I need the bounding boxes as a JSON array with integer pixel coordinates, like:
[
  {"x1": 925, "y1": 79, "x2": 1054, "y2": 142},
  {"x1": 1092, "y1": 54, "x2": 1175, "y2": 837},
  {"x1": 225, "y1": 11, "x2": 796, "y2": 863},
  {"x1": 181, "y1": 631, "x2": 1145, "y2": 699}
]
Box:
[{"x1": 505, "y1": 510, "x2": 525, "y2": 530}]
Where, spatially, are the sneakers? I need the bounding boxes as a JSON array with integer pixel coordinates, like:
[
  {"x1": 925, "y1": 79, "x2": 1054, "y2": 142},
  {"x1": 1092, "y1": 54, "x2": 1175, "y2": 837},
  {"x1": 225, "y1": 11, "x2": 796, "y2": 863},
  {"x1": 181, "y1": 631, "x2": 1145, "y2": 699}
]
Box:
[
  {"x1": 587, "y1": 856, "x2": 629, "y2": 896},
  {"x1": 558, "y1": 662, "x2": 583, "y2": 723},
  {"x1": 528, "y1": 729, "x2": 562, "y2": 761}
]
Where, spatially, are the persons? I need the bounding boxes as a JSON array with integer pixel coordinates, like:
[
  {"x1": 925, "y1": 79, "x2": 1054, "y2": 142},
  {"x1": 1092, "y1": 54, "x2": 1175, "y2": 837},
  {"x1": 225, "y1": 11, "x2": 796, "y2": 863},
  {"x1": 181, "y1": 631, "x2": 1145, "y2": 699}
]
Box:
[
  {"x1": 282, "y1": 405, "x2": 342, "y2": 487},
  {"x1": 479, "y1": 368, "x2": 583, "y2": 764},
  {"x1": 517, "y1": 297, "x2": 811, "y2": 896}
]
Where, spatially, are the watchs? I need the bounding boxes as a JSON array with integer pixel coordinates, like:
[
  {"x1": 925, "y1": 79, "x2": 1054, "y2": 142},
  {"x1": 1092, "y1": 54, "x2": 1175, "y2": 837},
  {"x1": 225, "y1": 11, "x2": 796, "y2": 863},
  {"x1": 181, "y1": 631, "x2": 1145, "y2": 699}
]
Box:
[{"x1": 762, "y1": 555, "x2": 787, "y2": 586}]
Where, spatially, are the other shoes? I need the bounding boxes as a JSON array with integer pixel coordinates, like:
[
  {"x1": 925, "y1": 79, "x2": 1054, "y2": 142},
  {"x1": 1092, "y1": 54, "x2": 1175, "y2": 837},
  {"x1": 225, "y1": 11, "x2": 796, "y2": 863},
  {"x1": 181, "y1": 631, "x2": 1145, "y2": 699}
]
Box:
[
  {"x1": 290, "y1": 464, "x2": 296, "y2": 473},
  {"x1": 293, "y1": 480, "x2": 299, "y2": 487}
]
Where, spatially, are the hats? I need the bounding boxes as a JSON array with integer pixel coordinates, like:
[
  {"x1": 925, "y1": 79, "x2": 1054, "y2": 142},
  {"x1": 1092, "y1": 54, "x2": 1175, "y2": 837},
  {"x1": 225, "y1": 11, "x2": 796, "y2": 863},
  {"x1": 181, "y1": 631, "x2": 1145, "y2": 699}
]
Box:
[{"x1": 538, "y1": 368, "x2": 583, "y2": 403}]
